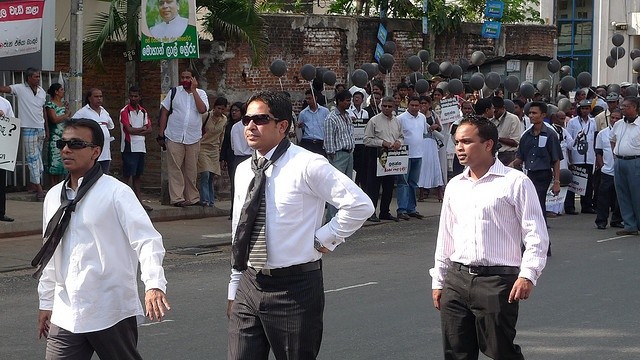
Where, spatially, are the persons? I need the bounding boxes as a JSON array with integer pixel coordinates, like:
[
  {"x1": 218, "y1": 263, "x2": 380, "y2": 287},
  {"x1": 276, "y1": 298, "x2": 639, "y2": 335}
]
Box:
[
  {"x1": 225, "y1": 88, "x2": 375, "y2": 360},
  {"x1": 328, "y1": 84, "x2": 347, "y2": 112},
  {"x1": 31, "y1": 117, "x2": 172, "y2": 360},
  {"x1": 3, "y1": 66, "x2": 51, "y2": 202},
  {"x1": 430, "y1": 88, "x2": 444, "y2": 104},
  {"x1": 196, "y1": 97, "x2": 229, "y2": 211},
  {"x1": 594, "y1": 107, "x2": 625, "y2": 231},
  {"x1": 414, "y1": 95, "x2": 443, "y2": 204},
  {"x1": 281, "y1": 90, "x2": 299, "y2": 145},
  {"x1": 532, "y1": 91, "x2": 542, "y2": 101},
  {"x1": 302, "y1": 78, "x2": 327, "y2": 113},
  {"x1": 348, "y1": 77, "x2": 371, "y2": 110},
  {"x1": 428, "y1": 116, "x2": 550, "y2": 360},
  {"x1": 300, "y1": 88, "x2": 328, "y2": 152},
  {"x1": 431, "y1": 102, "x2": 453, "y2": 202},
  {"x1": 472, "y1": 90, "x2": 480, "y2": 101},
  {"x1": 495, "y1": 89, "x2": 504, "y2": 99},
  {"x1": 218, "y1": 102, "x2": 245, "y2": 222},
  {"x1": 594, "y1": 92, "x2": 626, "y2": 132},
  {"x1": 395, "y1": 97, "x2": 429, "y2": 220},
  {"x1": 572, "y1": 90, "x2": 587, "y2": 106},
  {"x1": 0, "y1": 95, "x2": 18, "y2": 223},
  {"x1": 149, "y1": 0, "x2": 189, "y2": 44},
  {"x1": 448, "y1": 101, "x2": 475, "y2": 178},
  {"x1": 71, "y1": 87, "x2": 116, "y2": 176},
  {"x1": 397, "y1": 82, "x2": 410, "y2": 112},
  {"x1": 362, "y1": 92, "x2": 382, "y2": 120},
  {"x1": 324, "y1": 89, "x2": 354, "y2": 226},
  {"x1": 349, "y1": 90, "x2": 370, "y2": 195},
  {"x1": 484, "y1": 95, "x2": 523, "y2": 167},
  {"x1": 391, "y1": 91, "x2": 405, "y2": 117},
  {"x1": 507, "y1": 102, "x2": 564, "y2": 257},
  {"x1": 586, "y1": 81, "x2": 640, "y2": 99},
  {"x1": 407, "y1": 84, "x2": 416, "y2": 98},
  {"x1": 361, "y1": 95, "x2": 405, "y2": 223},
  {"x1": 565, "y1": 99, "x2": 601, "y2": 216},
  {"x1": 46, "y1": 81, "x2": 72, "y2": 189},
  {"x1": 547, "y1": 109, "x2": 585, "y2": 219},
  {"x1": 609, "y1": 95, "x2": 640, "y2": 237},
  {"x1": 465, "y1": 94, "x2": 477, "y2": 111},
  {"x1": 511, "y1": 99, "x2": 531, "y2": 132},
  {"x1": 230, "y1": 102, "x2": 253, "y2": 216},
  {"x1": 370, "y1": 83, "x2": 387, "y2": 111},
  {"x1": 470, "y1": 97, "x2": 499, "y2": 158},
  {"x1": 117, "y1": 84, "x2": 155, "y2": 212},
  {"x1": 157, "y1": 67, "x2": 211, "y2": 209}
]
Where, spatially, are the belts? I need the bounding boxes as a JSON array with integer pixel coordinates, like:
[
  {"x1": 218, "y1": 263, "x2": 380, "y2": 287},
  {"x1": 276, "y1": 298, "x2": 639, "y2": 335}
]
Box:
[
  {"x1": 615, "y1": 155, "x2": 640, "y2": 159},
  {"x1": 452, "y1": 263, "x2": 519, "y2": 276},
  {"x1": 245, "y1": 262, "x2": 322, "y2": 278}
]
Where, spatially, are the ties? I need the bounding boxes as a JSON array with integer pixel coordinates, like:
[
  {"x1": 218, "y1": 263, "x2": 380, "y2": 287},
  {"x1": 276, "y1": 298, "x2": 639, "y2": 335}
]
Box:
[{"x1": 248, "y1": 158, "x2": 267, "y2": 274}]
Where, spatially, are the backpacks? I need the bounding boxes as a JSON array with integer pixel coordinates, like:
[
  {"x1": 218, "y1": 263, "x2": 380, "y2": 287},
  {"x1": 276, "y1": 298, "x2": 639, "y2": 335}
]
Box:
[{"x1": 157, "y1": 86, "x2": 176, "y2": 130}]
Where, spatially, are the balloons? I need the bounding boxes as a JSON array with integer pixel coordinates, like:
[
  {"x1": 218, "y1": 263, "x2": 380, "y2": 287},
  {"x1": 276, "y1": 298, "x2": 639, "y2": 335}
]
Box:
[
  {"x1": 469, "y1": 75, "x2": 484, "y2": 90},
  {"x1": 449, "y1": 79, "x2": 464, "y2": 95},
  {"x1": 270, "y1": 59, "x2": 287, "y2": 77},
  {"x1": 485, "y1": 72, "x2": 500, "y2": 89},
  {"x1": 407, "y1": 56, "x2": 422, "y2": 71},
  {"x1": 560, "y1": 76, "x2": 576, "y2": 92},
  {"x1": 323, "y1": 70, "x2": 336, "y2": 86},
  {"x1": 471, "y1": 51, "x2": 486, "y2": 67},
  {"x1": 547, "y1": 59, "x2": 561, "y2": 74},
  {"x1": 611, "y1": 47, "x2": 623, "y2": 60},
  {"x1": 577, "y1": 71, "x2": 592, "y2": 88},
  {"x1": 522, "y1": 81, "x2": 532, "y2": 86},
  {"x1": 503, "y1": 99, "x2": 514, "y2": 113},
  {"x1": 458, "y1": 58, "x2": 469, "y2": 71},
  {"x1": 556, "y1": 95, "x2": 567, "y2": 104},
  {"x1": 360, "y1": 62, "x2": 375, "y2": 80},
  {"x1": 451, "y1": 65, "x2": 463, "y2": 78},
  {"x1": 431, "y1": 76, "x2": 442, "y2": 89},
  {"x1": 523, "y1": 102, "x2": 532, "y2": 116},
  {"x1": 504, "y1": 75, "x2": 518, "y2": 93},
  {"x1": 520, "y1": 83, "x2": 534, "y2": 98},
  {"x1": 418, "y1": 50, "x2": 430, "y2": 62},
  {"x1": 632, "y1": 57, "x2": 640, "y2": 72},
  {"x1": 371, "y1": 62, "x2": 379, "y2": 78},
  {"x1": 560, "y1": 65, "x2": 571, "y2": 80},
  {"x1": 300, "y1": 64, "x2": 317, "y2": 82},
  {"x1": 436, "y1": 81, "x2": 450, "y2": 97},
  {"x1": 427, "y1": 62, "x2": 440, "y2": 75},
  {"x1": 378, "y1": 62, "x2": 393, "y2": 74},
  {"x1": 410, "y1": 72, "x2": 424, "y2": 85},
  {"x1": 630, "y1": 48, "x2": 640, "y2": 60},
  {"x1": 558, "y1": 98, "x2": 572, "y2": 114},
  {"x1": 415, "y1": 79, "x2": 429, "y2": 93},
  {"x1": 472, "y1": 72, "x2": 484, "y2": 79},
  {"x1": 381, "y1": 53, "x2": 395, "y2": 68},
  {"x1": 606, "y1": 83, "x2": 621, "y2": 97},
  {"x1": 625, "y1": 86, "x2": 638, "y2": 98},
  {"x1": 606, "y1": 56, "x2": 616, "y2": 68},
  {"x1": 620, "y1": 47, "x2": 626, "y2": 57},
  {"x1": 383, "y1": 41, "x2": 397, "y2": 55},
  {"x1": 439, "y1": 61, "x2": 453, "y2": 77},
  {"x1": 537, "y1": 79, "x2": 550, "y2": 94},
  {"x1": 316, "y1": 69, "x2": 328, "y2": 82},
  {"x1": 612, "y1": 34, "x2": 624, "y2": 47},
  {"x1": 351, "y1": 69, "x2": 368, "y2": 88}
]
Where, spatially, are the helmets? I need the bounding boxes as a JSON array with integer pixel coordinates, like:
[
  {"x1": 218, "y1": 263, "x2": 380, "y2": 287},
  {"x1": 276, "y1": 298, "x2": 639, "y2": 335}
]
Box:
[
  {"x1": 579, "y1": 100, "x2": 591, "y2": 107},
  {"x1": 606, "y1": 92, "x2": 619, "y2": 102}
]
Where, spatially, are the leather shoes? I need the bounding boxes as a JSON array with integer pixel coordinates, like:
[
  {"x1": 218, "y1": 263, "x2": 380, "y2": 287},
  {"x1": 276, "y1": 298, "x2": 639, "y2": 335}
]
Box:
[
  {"x1": 381, "y1": 215, "x2": 398, "y2": 221},
  {"x1": 175, "y1": 200, "x2": 192, "y2": 206},
  {"x1": 410, "y1": 213, "x2": 424, "y2": 218},
  {"x1": 367, "y1": 215, "x2": 379, "y2": 221},
  {"x1": 1, "y1": 215, "x2": 14, "y2": 222},
  {"x1": 566, "y1": 210, "x2": 578, "y2": 215},
  {"x1": 616, "y1": 230, "x2": 639, "y2": 235},
  {"x1": 580, "y1": 209, "x2": 596, "y2": 215},
  {"x1": 610, "y1": 223, "x2": 623, "y2": 227},
  {"x1": 195, "y1": 201, "x2": 208, "y2": 206},
  {"x1": 599, "y1": 223, "x2": 605, "y2": 228},
  {"x1": 398, "y1": 213, "x2": 410, "y2": 219}
]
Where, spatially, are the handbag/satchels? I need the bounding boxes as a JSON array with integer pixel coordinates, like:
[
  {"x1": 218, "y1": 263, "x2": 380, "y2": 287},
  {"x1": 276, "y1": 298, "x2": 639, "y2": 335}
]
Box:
[{"x1": 431, "y1": 110, "x2": 444, "y2": 147}]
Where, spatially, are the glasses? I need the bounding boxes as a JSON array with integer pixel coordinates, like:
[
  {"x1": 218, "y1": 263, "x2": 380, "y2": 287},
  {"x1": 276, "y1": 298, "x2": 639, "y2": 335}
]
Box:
[
  {"x1": 56, "y1": 139, "x2": 95, "y2": 150},
  {"x1": 382, "y1": 105, "x2": 393, "y2": 108},
  {"x1": 242, "y1": 114, "x2": 283, "y2": 124},
  {"x1": 230, "y1": 109, "x2": 241, "y2": 112}
]
